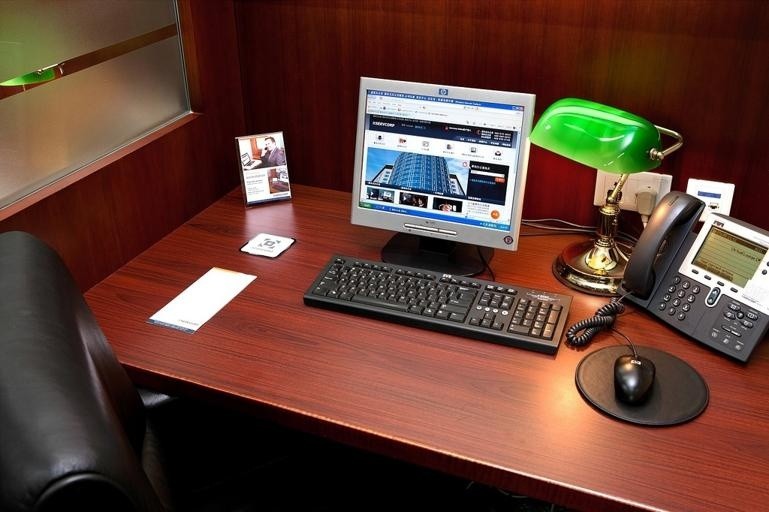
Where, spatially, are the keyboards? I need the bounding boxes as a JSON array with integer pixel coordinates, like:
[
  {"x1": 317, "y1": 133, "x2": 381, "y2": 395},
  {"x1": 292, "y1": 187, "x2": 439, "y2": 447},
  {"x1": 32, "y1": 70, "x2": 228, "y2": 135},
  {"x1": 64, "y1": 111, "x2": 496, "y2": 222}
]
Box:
[{"x1": 304, "y1": 254, "x2": 574, "y2": 355}]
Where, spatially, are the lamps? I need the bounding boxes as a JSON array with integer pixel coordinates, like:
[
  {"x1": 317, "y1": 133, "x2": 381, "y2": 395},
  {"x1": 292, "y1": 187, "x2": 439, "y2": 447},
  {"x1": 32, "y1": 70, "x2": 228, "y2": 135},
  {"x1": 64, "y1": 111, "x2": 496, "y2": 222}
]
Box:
[{"x1": 528, "y1": 97, "x2": 684, "y2": 297}]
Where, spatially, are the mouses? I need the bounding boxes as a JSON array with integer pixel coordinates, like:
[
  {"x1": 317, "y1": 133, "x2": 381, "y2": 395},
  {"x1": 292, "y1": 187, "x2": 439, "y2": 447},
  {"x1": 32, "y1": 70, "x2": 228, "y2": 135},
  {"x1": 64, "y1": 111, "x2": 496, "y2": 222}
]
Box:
[{"x1": 613, "y1": 353, "x2": 656, "y2": 408}]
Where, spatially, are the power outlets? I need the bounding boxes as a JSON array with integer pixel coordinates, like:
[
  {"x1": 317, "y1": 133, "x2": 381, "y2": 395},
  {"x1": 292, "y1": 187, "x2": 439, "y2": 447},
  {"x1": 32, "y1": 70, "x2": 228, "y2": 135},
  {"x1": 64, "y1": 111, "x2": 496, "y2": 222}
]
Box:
[{"x1": 593, "y1": 170, "x2": 673, "y2": 216}]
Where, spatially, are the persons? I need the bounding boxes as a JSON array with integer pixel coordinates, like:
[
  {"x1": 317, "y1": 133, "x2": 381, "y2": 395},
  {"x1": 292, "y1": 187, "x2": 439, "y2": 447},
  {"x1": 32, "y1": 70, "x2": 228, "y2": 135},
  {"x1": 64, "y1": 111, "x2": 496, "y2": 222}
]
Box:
[{"x1": 260, "y1": 136, "x2": 286, "y2": 167}]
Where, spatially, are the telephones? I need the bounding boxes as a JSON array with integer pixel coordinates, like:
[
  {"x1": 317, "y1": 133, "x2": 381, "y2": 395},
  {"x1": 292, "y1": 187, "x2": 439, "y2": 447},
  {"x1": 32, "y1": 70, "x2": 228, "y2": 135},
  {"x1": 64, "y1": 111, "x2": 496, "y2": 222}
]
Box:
[{"x1": 609, "y1": 191, "x2": 769, "y2": 365}]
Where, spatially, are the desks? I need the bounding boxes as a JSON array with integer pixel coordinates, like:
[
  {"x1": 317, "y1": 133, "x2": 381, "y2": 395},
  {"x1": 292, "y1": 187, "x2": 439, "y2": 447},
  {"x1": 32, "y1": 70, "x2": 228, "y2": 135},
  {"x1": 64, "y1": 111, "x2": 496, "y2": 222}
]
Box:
[{"x1": 83, "y1": 181, "x2": 769, "y2": 512}]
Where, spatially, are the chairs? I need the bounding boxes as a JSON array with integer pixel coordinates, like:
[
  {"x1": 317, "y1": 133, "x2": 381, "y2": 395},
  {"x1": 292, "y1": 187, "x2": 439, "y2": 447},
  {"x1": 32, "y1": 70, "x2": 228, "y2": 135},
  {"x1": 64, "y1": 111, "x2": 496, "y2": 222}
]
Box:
[{"x1": 0, "y1": 230, "x2": 490, "y2": 512}]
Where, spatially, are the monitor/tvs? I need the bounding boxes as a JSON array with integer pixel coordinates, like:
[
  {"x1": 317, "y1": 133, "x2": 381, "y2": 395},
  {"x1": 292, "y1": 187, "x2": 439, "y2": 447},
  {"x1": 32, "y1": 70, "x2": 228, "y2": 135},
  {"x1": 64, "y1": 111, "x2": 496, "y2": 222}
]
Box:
[{"x1": 350, "y1": 75, "x2": 538, "y2": 277}]
